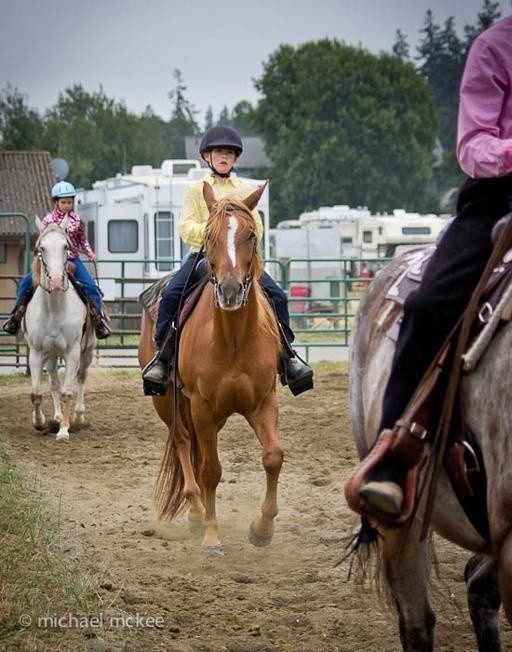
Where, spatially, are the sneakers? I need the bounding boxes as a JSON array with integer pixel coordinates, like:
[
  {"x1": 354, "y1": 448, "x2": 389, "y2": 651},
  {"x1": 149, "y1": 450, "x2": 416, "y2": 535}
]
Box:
[
  {"x1": 280, "y1": 357, "x2": 313, "y2": 386},
  {"x1": 359, "y1": 481, "x2": 403, "y2": 514},
  {"x1": 143, "y1": 353, "x2": 176, "y2": 384},
  {"x1": 95, "y1": 323, "x2": 109, "y2": 338},
  {"x1": 5, "y1": 318, "x2": 17, "y2": 333}
]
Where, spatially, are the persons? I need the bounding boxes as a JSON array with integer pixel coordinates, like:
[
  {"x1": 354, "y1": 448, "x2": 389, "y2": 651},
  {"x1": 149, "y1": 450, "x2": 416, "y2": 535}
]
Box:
[
  {"x1": 143, "y1": 124, "x2": 314, "y2": 388},
  {"x1": 360, "y1": 12, "x2": 511, "y2": 516},
  {"x1": 3, "y1": 180, "x2": 112, "y2": 340}
]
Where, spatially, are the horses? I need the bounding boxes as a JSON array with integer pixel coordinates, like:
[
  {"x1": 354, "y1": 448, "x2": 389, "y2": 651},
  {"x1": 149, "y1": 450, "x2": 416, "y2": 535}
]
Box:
[
  {"x1": 138, "y1": 178, "x2": 284, "y2": 559},
  {"x1": 20, "y1": 212, "x2": 98, "y2": 442},
  {"x1": 330, "y1": 244, "x2": 511, "y2": 652}
]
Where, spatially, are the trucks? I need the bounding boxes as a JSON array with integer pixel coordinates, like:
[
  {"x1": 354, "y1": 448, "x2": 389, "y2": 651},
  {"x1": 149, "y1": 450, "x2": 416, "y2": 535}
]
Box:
[
  {"x1": 265, "y1": 226, "x2": 344, "y2": 320},
  {"x1": 277, "y1": 204, "x2": 371, "y2": 278},
  {"x1": 73, "y1": 160, "x2": 270, "y2": 330},
  {"x1": 350, "y1": 207, "x2": 456, "y2": 278}
]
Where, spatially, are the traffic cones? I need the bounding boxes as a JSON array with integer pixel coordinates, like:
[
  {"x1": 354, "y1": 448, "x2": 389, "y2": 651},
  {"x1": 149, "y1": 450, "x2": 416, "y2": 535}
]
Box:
[{"x1": 355, "y1": 262, "x2": 370, "y2": 287}]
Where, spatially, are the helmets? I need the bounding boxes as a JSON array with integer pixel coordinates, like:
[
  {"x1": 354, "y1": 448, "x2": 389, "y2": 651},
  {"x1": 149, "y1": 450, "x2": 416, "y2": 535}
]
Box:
[
  {"x1": 51, "y1": 181, "x2": 76, "y2": 198},
  {"x1": 199, "y1": 125, "x2": 242, "y2": 156}
]
